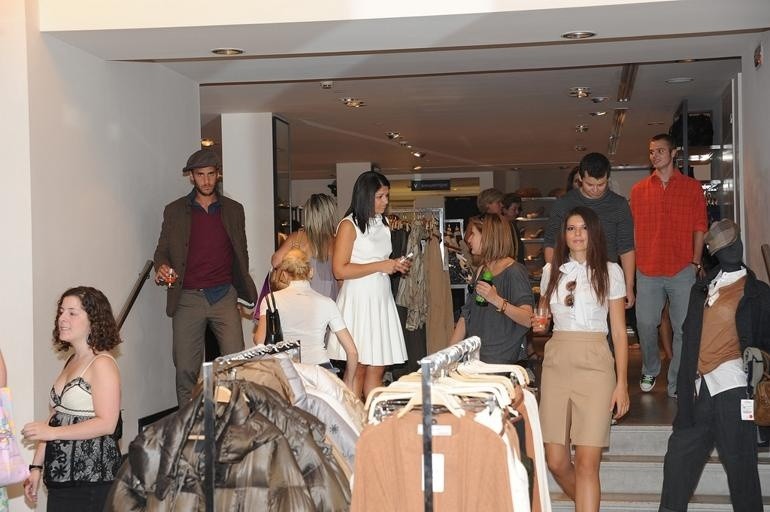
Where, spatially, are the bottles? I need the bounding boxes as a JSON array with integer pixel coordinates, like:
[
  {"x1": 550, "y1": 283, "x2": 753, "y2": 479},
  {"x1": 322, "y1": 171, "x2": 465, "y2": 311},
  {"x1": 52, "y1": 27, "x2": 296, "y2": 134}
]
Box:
[{"x1": 475, "y1": 272, "x2": 492, "y2": 306}]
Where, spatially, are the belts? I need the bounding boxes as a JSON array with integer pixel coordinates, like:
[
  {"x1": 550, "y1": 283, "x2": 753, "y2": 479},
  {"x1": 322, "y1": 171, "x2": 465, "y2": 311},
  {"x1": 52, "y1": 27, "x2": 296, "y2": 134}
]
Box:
[{"x1": 743, "y1": 347, "x2": 764, "y2": 395}]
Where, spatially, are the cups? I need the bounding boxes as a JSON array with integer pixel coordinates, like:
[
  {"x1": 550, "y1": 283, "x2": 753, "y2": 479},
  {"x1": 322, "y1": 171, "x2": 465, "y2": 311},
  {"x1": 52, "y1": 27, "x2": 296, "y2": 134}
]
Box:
[{"x1": 533, "y1": 306, "x2": 549, "y2": 332}]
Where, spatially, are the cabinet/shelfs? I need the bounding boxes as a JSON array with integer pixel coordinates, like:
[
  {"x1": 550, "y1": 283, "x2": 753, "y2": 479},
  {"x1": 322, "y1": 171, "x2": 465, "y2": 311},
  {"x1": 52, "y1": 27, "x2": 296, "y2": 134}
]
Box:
[{"x1": 514, "y1": 196, "x2": 558, "y2": 297}]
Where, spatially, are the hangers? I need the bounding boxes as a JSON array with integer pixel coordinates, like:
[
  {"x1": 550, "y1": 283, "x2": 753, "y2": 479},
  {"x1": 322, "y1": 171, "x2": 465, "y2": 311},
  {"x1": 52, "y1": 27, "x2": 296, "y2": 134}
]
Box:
[
  {"x1": 363, "y1": 337, "x2": 530, "y2": 418},
  {"x1": 207, "y1": 333, "x2": 300, "y2": 382},
  {"x1": 702, "y1": 192, "x2": 720, "y2": 206},
  {"x1": 389, "y1": 209, "x2": 441, "y2": 230}
]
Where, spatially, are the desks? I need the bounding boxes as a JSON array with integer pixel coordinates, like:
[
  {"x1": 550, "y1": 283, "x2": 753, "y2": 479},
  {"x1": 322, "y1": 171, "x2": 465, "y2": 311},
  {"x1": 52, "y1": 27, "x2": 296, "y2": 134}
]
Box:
[{"x1": 449, "y1": 284, "x2": 469, "y2": 305}]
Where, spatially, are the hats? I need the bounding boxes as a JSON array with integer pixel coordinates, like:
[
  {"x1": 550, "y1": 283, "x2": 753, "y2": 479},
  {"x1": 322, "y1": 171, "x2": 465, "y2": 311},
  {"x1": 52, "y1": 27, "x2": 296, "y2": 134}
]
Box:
[
  {"x1": 703, "y1": 218, "x2": 740, "y2": 256},
  {"x1": 183, "y1": 149, "x2": 220, "y2": 172}
]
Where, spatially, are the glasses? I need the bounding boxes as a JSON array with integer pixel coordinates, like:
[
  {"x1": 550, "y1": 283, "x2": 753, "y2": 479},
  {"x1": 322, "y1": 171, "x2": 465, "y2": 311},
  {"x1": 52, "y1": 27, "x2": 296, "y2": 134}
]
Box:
[{"x1": 564, "y1": 281, "x2": 576, "y2": 308}]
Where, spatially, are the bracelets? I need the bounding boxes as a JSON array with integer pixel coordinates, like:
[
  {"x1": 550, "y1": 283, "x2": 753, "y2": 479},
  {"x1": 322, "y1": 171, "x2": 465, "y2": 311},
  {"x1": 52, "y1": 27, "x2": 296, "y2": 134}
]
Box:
[
  {"x1": 29, "y1": 464, "x2": 43, "y2": 471},
  {"x1": 692, "y1": 262, "x2": 702, "y2": 270}
]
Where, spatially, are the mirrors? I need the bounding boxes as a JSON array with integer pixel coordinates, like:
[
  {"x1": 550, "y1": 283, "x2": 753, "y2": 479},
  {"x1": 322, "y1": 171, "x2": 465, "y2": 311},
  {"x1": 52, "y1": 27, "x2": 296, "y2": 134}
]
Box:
[
  {"x1": 721, "y1": 79, "x2": 736, "y2": 226},
  {"x1": 272, "y1": 116, "x2": 293, "y2": 253}
]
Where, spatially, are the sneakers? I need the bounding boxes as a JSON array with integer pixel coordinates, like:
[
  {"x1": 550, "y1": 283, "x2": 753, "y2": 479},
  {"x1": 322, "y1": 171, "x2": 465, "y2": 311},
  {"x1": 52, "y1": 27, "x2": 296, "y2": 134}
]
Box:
[{"x1": 640, "y1": 374, "x2": 656, "y2": 392}]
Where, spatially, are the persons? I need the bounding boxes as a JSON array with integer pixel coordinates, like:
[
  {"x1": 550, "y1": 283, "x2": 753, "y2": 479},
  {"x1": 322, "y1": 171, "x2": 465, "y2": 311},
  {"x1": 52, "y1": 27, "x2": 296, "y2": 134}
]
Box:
[
  {"x1": 529, "y1": 207, "x2": 632, "y2": 512},
  {"x1": 152, "y1": 149, "x2": 258, "y2": 415},
  {"x1": 447, "y1": 153, "x2": 673, "y2": 425},
  {"x1": 270, "y1": 193, "x2": 344, "y2": 307},
  {"x1": 252, "y1": 248, "x2": 359, "y2": 395},
  {"x1": 1, "y1": 348, "x2": 10, "y2": 512},
  {"x1": 628, "y1": 133, "x2": 709, "y2": 400},
  {"x1": 324, "y1": 170, "x2": 414, "y2": 403},
  {"x1": 657, "y1": 219, "x2": 769, "y2": 512},
  {"x1": 22, "y1": 286, "x2": 125, "y2": 512}
]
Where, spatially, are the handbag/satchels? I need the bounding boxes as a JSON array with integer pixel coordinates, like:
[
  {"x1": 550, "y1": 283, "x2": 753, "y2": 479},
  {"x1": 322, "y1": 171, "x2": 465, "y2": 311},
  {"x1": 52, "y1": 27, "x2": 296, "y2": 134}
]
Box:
[
  {"x1": 752, "y1": 350, "x2": 770, "y2": 426},
  {"x1": 265, "y1": 290, "x2": 283, "y2": 346}
]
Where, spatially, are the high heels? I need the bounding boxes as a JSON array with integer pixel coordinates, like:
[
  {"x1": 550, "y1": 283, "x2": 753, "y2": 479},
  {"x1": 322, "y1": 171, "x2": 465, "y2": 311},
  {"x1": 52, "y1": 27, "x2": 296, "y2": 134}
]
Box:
[{"x1": 527, "y1": 206, "x2": 544, "y2": 279}]
